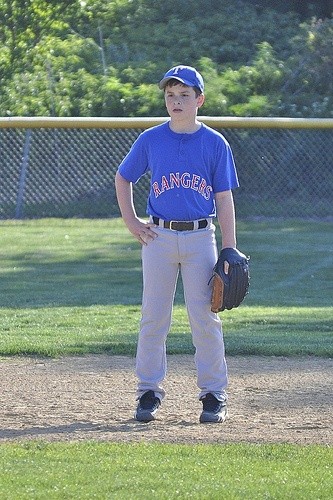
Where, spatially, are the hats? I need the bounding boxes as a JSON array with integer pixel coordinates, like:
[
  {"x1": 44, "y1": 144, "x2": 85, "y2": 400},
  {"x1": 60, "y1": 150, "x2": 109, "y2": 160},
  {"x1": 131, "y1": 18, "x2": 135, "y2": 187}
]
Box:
[{"x1": 159, "y1": 65, "x2": 204, "y2": 93}]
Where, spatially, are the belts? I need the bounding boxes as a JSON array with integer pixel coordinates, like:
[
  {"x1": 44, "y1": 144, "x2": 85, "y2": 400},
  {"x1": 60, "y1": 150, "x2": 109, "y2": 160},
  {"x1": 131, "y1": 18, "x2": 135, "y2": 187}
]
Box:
[{"x1": 152, "y1": 216, "x2": 208, "y2": 232}]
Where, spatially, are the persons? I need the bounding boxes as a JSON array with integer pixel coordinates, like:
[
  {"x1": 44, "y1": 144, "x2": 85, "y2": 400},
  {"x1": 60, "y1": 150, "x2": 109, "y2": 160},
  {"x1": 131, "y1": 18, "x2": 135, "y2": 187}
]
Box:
[{"x1": 115, "y1": 66, "x2": 238, "y2": 424}]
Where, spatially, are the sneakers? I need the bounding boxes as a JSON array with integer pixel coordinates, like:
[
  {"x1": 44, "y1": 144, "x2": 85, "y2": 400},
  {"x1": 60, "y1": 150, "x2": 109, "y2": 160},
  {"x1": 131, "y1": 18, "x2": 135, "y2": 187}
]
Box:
[
  {"x1": 199, "y1": 393, "x2": 230, "y2": 423},
  {"x1": 136, "y1": 390, "x2": 162, "y2": 421}
]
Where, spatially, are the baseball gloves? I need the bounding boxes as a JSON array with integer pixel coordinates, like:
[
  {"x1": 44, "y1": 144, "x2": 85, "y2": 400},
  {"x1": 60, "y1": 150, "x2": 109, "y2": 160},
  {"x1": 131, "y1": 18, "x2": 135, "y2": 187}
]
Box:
[{"x1": 210, "y1": 247, "x2": 250, "y2": 314}]
